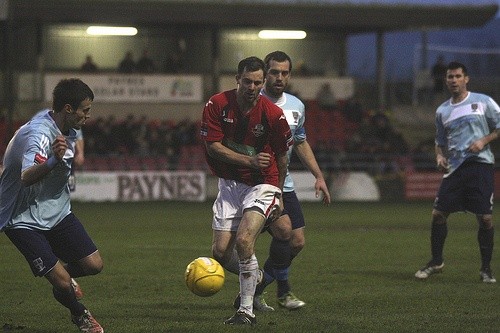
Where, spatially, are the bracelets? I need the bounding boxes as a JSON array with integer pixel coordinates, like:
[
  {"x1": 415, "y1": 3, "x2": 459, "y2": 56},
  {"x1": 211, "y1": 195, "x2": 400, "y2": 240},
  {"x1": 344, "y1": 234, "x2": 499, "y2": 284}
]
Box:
[{"x1": 46, "y1": 155, "x2": 58, "y2": 170}]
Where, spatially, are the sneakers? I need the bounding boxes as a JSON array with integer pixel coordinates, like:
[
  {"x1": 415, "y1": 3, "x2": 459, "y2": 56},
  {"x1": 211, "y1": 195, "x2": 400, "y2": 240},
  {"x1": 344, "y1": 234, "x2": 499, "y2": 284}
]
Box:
[
  {"x1": 68, "y1": 274, "x2": 83, "y2": 302},
  {"x1": 71, "y1": 308, "x2": 104, "y2": 333},
  {"x1": 224, "y1": 310, "x2": 257, "y2": 326},
  {"x1": 252, "y1": 295, "x2": 275, "y2": 312},
  {"x1": 414, "y1": 261, "x2": 444, "y2": 278},
  {"x1": 276, "y1": 291, "x2": 305, "y2": 310},
  {"x1": 233, "y1": 269, "x2": 265, "y2": 309},
  {"x1": 478, "y1": 268, "x2": 496, "y2": 282}
]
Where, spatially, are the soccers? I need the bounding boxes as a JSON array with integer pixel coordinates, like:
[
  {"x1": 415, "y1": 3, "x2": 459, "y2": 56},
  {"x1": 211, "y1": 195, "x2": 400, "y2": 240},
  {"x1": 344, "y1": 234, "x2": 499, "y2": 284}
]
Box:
[{"x1": 185, "y1": 256, "x2": 225, "y2": 298}]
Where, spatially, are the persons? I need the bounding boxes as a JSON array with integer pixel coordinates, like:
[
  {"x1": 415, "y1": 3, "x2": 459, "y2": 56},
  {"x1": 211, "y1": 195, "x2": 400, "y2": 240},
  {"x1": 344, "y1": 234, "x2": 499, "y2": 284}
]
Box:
[
  {"x1": 414, "y1": 62, "x2": 500, "y2": 283},
  {"x1": 252, "y1": 51, "x2": 331, "y2": 311},
  {"x1": 0, "y1": 48, "x2": 444, "y2": 172},
  {"x1": 0, "y1": 79, "x2": 103, "y2": 333},
  {"x1": 199, "y1": 56, "x2": 292, "y2": 324}
]
